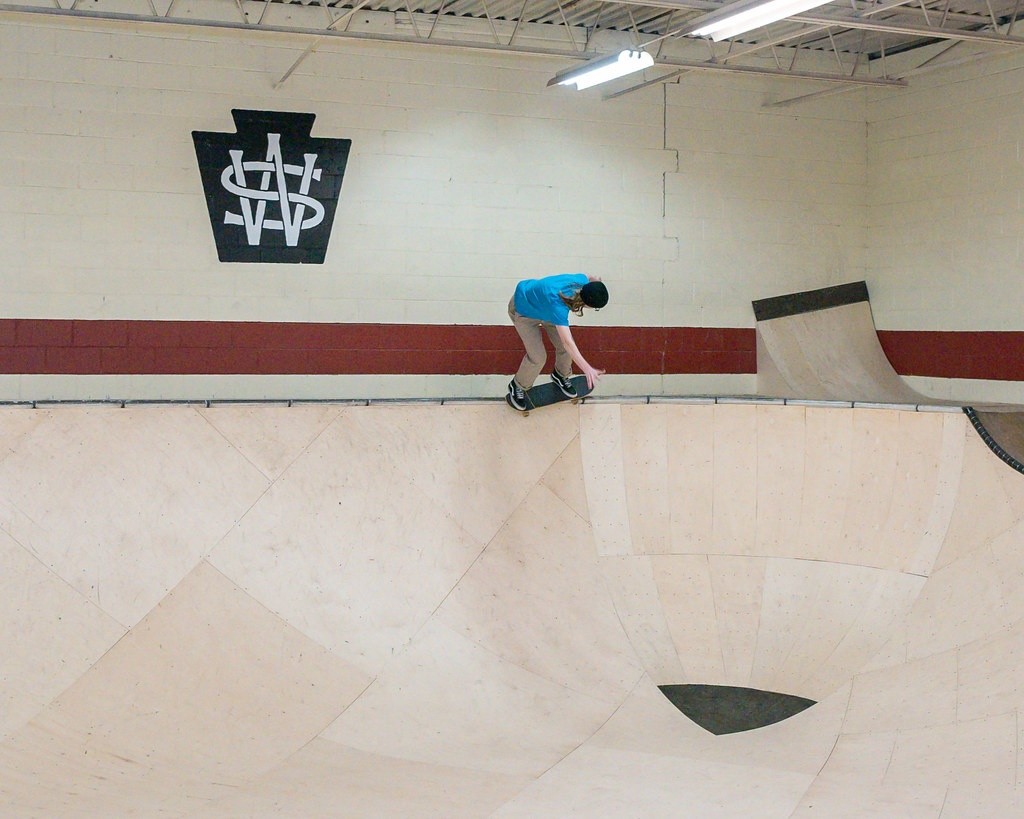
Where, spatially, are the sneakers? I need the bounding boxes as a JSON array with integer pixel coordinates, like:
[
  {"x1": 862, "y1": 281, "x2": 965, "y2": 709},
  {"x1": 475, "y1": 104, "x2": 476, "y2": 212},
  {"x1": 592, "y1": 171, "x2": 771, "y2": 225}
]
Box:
[
  {"x1": 508, "y1": 379, "x2": 526, "y2": 410},
  {"x1": 550, "y1": 370, "x2": 577, "y2": 398}
]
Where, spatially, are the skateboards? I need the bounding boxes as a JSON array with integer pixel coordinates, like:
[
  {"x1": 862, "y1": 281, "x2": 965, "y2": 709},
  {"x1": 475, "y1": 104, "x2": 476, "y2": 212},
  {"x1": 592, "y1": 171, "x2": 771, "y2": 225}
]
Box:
[{"x1": 504, "y1": 375, "x2": 595, "y2": 418}]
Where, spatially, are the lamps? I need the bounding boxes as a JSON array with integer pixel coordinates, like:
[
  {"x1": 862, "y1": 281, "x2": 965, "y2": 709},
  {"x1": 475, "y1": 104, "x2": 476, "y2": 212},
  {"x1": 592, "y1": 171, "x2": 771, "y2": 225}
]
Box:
[
  {"x1": 675, "y1": 0, "x2": 833, "y2": 44},
  {"x1": 545, "y1": 49, "x2": 653, "y2": 91}
]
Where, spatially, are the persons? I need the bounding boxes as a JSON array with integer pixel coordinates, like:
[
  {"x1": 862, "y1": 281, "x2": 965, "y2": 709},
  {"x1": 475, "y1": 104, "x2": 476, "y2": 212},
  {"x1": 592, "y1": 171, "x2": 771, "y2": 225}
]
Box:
[{"x1": 508, "y1": 273, "x2": 609, "y2": 411}]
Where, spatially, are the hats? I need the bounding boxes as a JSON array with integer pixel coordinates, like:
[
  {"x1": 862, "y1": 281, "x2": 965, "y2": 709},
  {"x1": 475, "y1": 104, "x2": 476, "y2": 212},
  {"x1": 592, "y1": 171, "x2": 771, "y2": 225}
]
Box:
[{"x1": 581, "y1": 281, "x2": 608, "y2": 308}]
